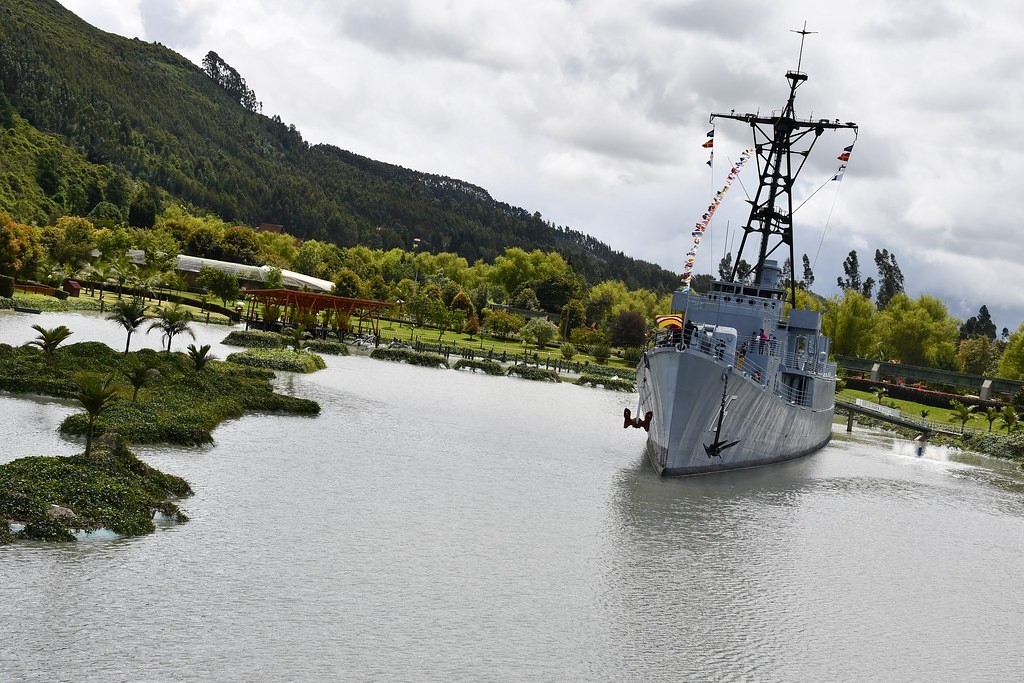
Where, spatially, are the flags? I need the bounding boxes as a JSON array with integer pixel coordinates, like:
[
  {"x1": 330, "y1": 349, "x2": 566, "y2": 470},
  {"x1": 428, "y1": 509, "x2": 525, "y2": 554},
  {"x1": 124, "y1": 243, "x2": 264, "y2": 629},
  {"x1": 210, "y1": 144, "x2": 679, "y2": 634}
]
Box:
[{"x1": 655, "y1": 314, "x2": 683, "y2": 329}]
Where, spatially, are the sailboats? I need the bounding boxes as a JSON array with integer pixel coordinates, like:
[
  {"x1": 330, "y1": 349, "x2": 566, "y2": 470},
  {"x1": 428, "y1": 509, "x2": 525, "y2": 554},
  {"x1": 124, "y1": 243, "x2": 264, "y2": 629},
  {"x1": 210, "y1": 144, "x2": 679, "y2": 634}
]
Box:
[{"x1": 634, "y1": 19, "x2": 843, "y2": 481}]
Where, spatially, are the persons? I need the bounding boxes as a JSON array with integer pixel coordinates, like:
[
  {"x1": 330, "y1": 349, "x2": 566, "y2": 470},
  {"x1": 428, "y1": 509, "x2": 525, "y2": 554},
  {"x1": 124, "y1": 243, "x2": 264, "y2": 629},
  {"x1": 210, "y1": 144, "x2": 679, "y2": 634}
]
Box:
[
  {"x1": 645, "y1": 320, "x2": 726, "y2": 361},
  {"x1": 735, "y1": 328, "x2": 777, "y2": 382}
]
